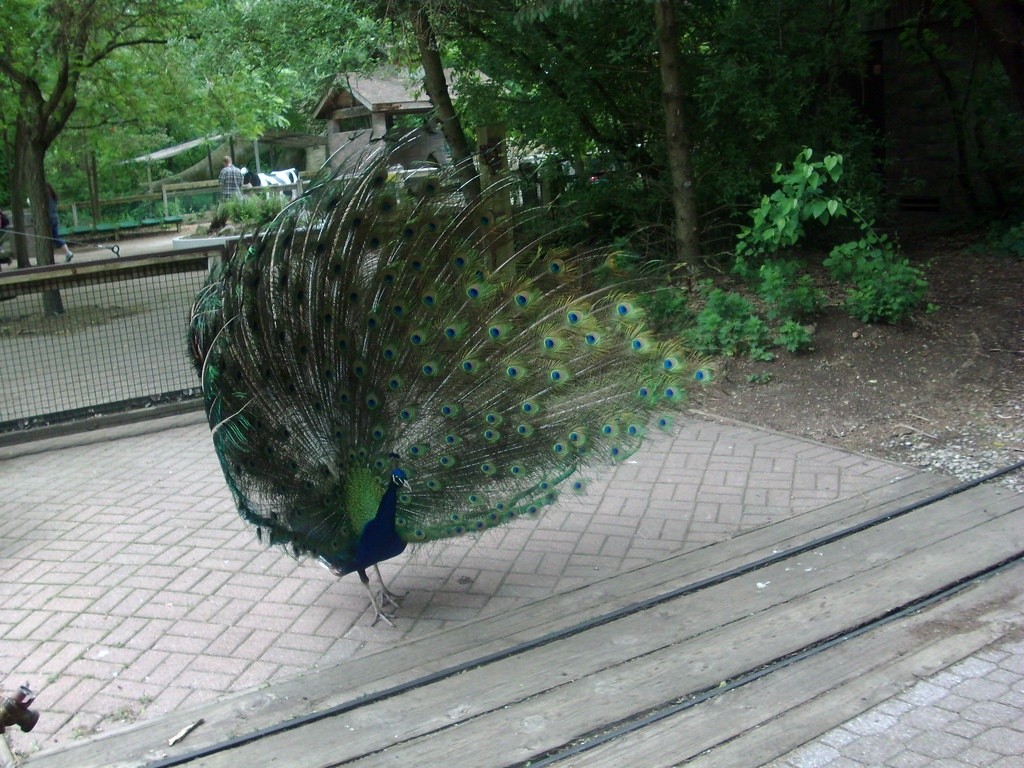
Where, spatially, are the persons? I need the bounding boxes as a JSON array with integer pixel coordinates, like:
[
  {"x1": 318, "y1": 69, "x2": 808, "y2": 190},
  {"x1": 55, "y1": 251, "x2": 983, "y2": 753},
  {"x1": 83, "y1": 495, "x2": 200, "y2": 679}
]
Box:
[
  {"x1": 217, "y1": 156, "x2": 244, "y2": 214},
  {"x1": 0, "y1": 212, "x2": 12, "y2": 272},
  {"x1": 27, "y1": 181, "x2": 74, "y2": 263}
]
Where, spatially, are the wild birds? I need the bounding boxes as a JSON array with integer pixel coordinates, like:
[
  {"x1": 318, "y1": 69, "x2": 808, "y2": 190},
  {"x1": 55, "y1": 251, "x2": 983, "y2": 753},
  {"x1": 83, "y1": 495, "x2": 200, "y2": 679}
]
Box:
[{"x1": 184, "y1": 104, "x2": 731, "y2": 631}]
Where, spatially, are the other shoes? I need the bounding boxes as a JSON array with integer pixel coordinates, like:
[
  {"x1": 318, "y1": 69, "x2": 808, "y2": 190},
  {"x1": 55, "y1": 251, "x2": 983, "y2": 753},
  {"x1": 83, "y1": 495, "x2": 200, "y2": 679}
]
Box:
[{"x1": 66, "y1": 252, "x2": 73, "y2": 262}]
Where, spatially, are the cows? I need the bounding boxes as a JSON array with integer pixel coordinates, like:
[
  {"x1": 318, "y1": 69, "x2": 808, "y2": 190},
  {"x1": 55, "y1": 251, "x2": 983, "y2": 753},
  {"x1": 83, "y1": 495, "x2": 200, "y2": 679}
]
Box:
[{"x1": 243, "y1": 167, "x2": 299, "y2": 186}]
